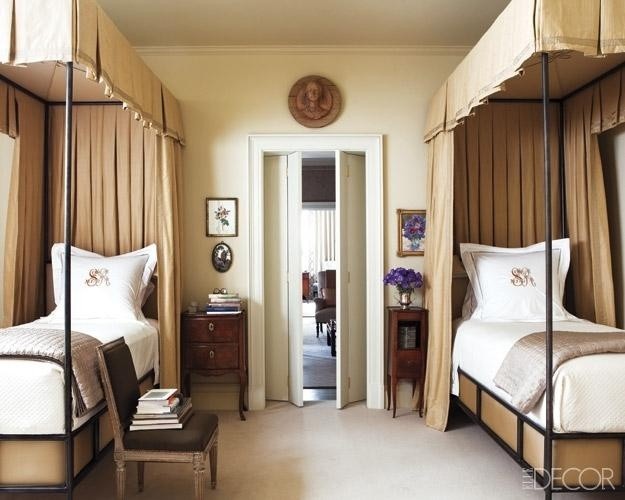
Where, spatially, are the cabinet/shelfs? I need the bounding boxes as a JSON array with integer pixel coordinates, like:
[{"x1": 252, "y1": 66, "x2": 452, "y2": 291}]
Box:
[{"x1": 302, "y1": 272, "x2": 311, "y2": 302}]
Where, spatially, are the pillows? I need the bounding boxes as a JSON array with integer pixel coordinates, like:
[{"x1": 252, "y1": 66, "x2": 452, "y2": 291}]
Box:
[
  {"x1": 460, "y1": 237, "x2": 583, "y2": 321},
  {"x1": 39, "y1": 241, "x2": 155, "y2": 326}
]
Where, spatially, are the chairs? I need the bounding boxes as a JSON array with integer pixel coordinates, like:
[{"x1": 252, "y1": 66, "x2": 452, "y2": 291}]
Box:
[
  {"x1": 314, "y1": 270, "x2": 336, "y2": 338},
  {"x1": 95, "y1": 336, "x2": 219, "y2": 500}
]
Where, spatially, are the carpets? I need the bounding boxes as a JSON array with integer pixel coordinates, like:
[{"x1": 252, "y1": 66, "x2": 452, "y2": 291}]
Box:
[{"x1": 303, "y1": 356, "x2": 336, "y2": 388}]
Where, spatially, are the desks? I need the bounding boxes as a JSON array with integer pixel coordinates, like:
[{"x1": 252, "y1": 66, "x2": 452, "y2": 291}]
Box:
[{"x1": 327, "y1": 319, "x2": 336, "y2": 357}]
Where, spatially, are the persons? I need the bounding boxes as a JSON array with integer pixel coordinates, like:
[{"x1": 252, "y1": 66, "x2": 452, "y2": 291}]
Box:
[{"x1": 299, "y1": 81, "x2": 329, "y2": 119}]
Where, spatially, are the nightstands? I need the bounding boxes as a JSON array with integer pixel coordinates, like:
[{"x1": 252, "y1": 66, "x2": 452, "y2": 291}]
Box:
[
  {"x1": 180, "y1": 310, "x2": 248, "y2": 421},
  {"x1": 386, "y1": 306, "x2": 429, "y2": 418}
]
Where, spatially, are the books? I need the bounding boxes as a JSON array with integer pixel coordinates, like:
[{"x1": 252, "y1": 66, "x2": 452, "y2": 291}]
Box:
[
  {"x1": 205, "y1": 293, "x2": 242, "y2": 315},
  {"x1": 128, "y1": 388, "x2": 195, "y2": 431}
]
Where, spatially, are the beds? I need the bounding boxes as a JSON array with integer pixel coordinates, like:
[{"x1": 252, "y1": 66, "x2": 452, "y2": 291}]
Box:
[
  {"x1": 1, "y1": 0, "x2": 186, "y2": 500},
  {"x1": 410, "y1": 0, "x2": 625, "y2": 500}
]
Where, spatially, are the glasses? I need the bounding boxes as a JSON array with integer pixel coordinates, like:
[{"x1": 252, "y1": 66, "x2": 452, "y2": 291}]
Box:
[{"x1": 213, "y1": 287, "x2": 227, "y2": 294}]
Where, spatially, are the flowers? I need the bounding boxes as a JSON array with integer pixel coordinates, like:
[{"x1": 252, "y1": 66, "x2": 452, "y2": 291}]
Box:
[{"x1": 383, "y1": 267, "x2": 423, "y2": 293}]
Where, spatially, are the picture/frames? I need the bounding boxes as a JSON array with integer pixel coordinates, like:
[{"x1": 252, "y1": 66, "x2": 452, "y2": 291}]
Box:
[
  {"x1": 205, "y1": 197, "x2": 239, "y2": 237},
  {"x1": 397, "y1": 209, "x2": 426, "y2": 258}
]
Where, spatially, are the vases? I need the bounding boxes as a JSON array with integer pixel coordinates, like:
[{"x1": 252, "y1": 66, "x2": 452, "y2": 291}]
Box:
[{"x1": 399, "y1": 293, "x2": 411, "y2": 310}]
